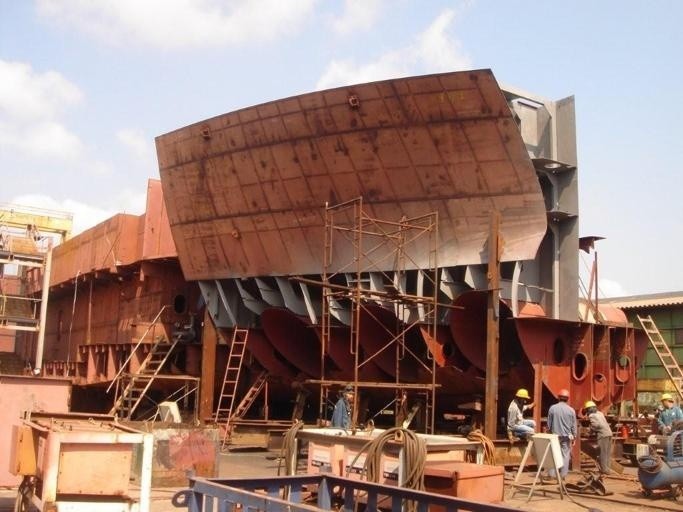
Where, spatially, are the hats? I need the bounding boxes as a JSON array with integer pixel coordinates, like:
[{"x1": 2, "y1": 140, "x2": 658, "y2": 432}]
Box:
[{"x1": 344, "y1": 383, "x2": 356, "y2": 394}]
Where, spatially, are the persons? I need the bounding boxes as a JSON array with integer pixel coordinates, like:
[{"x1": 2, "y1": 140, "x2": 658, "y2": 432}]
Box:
[
  {"x1": 543, "y1": 390, "x2": 577, "y2": 480},
  {"x1": 331, "y1": 384, "x2": 355, "y2": 428},
  {"x1": 655, "y1": 394, "x2": 683, "y2": 433},
  {"x1": 506, "y1": 388, "x2": 537, "y2": 437},
  {"x1": 582, "y1": 401, "x2": 613, "y2": 474}
]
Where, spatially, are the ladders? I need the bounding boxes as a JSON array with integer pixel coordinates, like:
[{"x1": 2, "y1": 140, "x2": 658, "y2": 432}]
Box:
[
  {"x1": 637, "y1": 314, "x2": 683, "y2": 400},
  {"x1": 227, "y1": 369, "x2": 268, "y2": 430},
  {"x1": 214, "y1": 324, "x2": 249, "y2": 451}
]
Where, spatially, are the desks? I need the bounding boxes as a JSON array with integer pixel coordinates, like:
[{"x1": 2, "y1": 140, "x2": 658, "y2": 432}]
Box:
[{"x1": 294, "y1": 428, "x2": 482, "y2": 508}]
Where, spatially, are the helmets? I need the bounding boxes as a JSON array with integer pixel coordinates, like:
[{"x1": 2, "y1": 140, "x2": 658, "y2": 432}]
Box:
[
  {"x1": 584, "y1": 400, "x2": 596, "y2": 410},
  {"x1": 660, "y1": 393, "x2": 672, "y2": 402},
  {"x1": 515, "y1": 389, "x2": 530, "y2": 400},
  {"x1": 558, "y1": 389, "x2": 571, "y2": 399}
]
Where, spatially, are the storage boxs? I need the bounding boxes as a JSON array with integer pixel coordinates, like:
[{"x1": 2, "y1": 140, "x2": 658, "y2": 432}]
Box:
[{"x1": 417, "y1": 460, "x2": 504, "y2": 503}]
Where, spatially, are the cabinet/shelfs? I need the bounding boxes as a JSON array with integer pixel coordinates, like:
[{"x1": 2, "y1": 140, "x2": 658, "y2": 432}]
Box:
[{"x1": 19, "y1": 410, "x2": 153, "y2": 512}]
